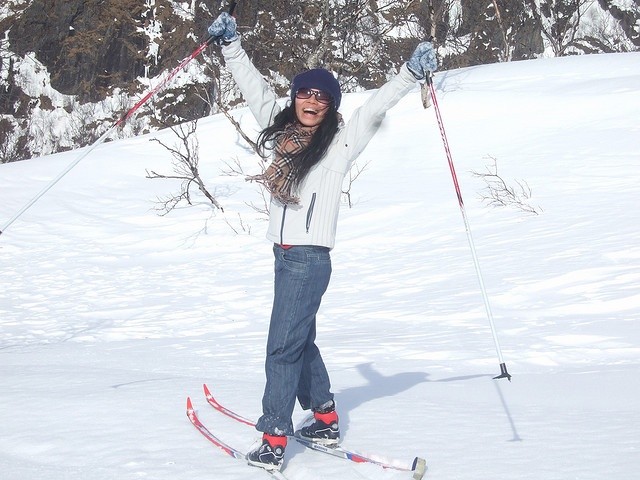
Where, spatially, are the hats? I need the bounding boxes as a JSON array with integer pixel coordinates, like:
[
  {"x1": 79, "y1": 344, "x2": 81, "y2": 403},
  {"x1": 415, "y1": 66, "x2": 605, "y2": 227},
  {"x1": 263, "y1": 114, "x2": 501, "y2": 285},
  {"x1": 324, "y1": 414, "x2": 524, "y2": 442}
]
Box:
[{"x1": 291, "y1": 69, "x2": 341, "y2": 106}]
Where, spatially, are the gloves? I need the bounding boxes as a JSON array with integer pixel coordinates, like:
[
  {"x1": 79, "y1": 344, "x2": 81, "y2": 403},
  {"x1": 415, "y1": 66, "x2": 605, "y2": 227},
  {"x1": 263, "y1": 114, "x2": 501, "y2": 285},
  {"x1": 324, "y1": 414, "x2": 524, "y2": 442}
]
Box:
[
  {"x1": 408, "y1": 42, "x2": 438, "y2": 81},
  {"x1": 208, "y1": 11, "x2": 238, "y2": 40}
]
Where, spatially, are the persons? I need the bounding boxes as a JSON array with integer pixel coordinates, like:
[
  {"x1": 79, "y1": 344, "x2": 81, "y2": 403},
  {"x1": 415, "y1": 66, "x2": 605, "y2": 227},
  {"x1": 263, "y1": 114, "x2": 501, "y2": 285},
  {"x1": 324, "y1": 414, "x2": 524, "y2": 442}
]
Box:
[{"x1": 206, "y1": 12, "x2": 438, "y2": 469}]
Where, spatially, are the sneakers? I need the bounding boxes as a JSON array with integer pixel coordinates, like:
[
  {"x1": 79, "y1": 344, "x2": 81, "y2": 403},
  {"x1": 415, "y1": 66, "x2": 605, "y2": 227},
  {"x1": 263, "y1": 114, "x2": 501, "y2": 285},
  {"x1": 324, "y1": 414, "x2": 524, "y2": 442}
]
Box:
[
  {"x1": 296, "y1": 421, "x2": 340, "y2": 446},
  {"x1": 245, "y1": 442, "x2": 285, "y2": 470}
]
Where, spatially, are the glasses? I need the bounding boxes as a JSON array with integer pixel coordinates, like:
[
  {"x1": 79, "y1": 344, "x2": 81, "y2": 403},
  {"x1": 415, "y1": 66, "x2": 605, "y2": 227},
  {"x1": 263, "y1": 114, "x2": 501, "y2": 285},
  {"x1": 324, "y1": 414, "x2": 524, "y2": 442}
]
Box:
[{"x1": 296, "y1": 86, "x2": 334, "y2": 104}]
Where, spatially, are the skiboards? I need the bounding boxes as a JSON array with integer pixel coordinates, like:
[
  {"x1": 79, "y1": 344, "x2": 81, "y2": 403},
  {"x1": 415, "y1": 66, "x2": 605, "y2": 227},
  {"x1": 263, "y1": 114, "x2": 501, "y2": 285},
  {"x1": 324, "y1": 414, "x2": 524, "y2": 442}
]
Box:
[{"x1": 187, "y1": 384, "x2": 410, "y2": 480}]
